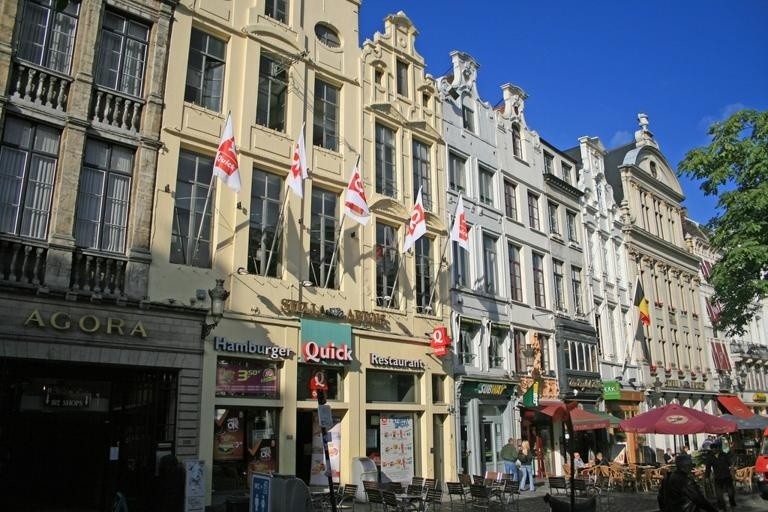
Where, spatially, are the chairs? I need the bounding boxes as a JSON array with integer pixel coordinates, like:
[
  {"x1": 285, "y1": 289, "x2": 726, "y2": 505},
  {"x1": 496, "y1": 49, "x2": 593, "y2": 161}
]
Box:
[{"x1": 309, "y1": 460, "x2": 759, "y2": 511}]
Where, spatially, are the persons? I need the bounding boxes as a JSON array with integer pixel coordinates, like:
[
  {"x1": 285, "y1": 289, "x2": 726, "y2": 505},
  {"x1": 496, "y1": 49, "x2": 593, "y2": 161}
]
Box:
[
  {"x1": 658, "y1": 435, "x2": 740, "y2": 511},
  {"x1": 574, "y1": 453, "x2": 585, "y2": 477},
  {"x1": 518, "y1": 440, "x2": 536, "y2": 492},
  {"x1": 589, "y1": 452, "x2": 610, "y2": 469},
  {"x1": 500, "y1": 438, "x2": 519, "y2": 485}
]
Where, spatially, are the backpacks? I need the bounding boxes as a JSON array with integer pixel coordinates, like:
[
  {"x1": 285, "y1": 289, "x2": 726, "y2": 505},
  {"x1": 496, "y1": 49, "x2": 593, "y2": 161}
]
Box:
[{"x1": 659, "y1": 470, "x2": 687, "y2": 511}]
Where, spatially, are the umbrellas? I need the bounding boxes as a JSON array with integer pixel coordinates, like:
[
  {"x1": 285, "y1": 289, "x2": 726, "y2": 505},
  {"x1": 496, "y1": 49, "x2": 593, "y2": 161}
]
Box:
[
  {"x1": 559, "y1": 406, "x2": 622, "y2": 432},
  {"x1": 722, "y1": 412, "x2": 768, "y2": 429},
  {"x1": 620, "y1": 402, "x2": 736, "y2": 463}
]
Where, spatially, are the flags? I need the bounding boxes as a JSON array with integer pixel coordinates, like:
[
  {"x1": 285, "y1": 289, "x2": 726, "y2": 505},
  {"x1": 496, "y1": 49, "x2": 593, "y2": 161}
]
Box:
[
  {"x1": 403, "y1": 184, "x2": 427, "y2": 253},
  {"x1": 285, "y1": 123, "x2": 309, "y2": 200},
  {"x1": 634, "y1": 278, "x2": 650, "y2": 323},
  {"x1": 449, "y1": 194, "x2": 471, "y2": 254},
  {"x1": 343, "y1": 159, "x2": 370, "y2": 227},
  {"x1": 211, "y1": 111, "x2": 241, "y2": 190}
]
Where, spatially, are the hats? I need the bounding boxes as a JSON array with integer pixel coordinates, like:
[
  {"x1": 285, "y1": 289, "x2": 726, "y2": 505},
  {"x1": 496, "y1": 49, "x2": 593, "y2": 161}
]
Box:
[{"x1": 709, "y1": 439, "x2": 722, "y2": 446}]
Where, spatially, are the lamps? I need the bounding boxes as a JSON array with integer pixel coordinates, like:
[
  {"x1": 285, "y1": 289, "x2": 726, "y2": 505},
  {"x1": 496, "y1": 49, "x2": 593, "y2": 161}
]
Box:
[
  {"x1": 414, "y1": 305, "x2": 433, "y2": 310},
  {"x1": 301, "y1": 279, "x2": 312, "y2": 287},
  {"x1": 736, "y1": 367, "x2": 747, "y2": 386},
  {"x1": 370, "y1": 295, "x2": 392, "y2": 302},
  {"x1": 226, "y1": 266, "x2": 248, "y2": 280},
  {"x1": 522, "y1": 343, "x2": 538, "y2": 367},
  {"x1": 202, "y1": 278, "x2": 233, "y2": 340}
]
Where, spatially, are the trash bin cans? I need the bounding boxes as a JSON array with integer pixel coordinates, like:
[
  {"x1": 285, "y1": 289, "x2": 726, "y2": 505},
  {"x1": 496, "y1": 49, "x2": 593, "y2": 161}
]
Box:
[
  {"x1": 251, "y1": 471, "x2": 307, "y2": 512},
  {"x1": 351, "y1": 456, "x2": 379, "y2": 504}
]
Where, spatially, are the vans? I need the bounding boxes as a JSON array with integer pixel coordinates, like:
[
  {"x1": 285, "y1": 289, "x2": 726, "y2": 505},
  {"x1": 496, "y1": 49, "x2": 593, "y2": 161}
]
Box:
[{"x1": 753, "y1": 426, "x2": 767, "y2": 500}]
